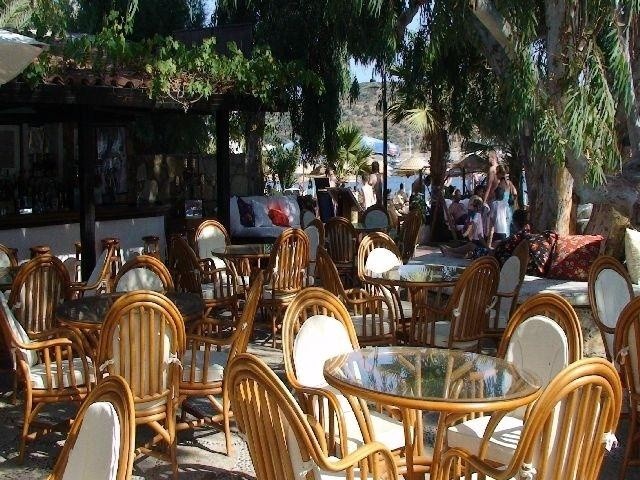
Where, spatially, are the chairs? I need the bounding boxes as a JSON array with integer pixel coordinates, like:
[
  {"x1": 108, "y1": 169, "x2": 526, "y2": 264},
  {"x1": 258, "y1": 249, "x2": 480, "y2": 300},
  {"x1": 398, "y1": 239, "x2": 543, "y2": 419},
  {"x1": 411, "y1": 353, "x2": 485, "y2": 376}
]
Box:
[
  {"x1": 419, "y1": 255, "x2": 500, "y2": 352},
  {"x1": 279, "y1": 282, "x2": 417, "y2": 480},
  {"x1": 195, "y1": 219, "x2": 231, "y2": 283},
  {"x1": 220, "y1": 353, "x2": 402, "y2": 480},
  {"x1": 237, "y1": 228, "x2": 309, "y2": 338},
  {"x1": 358, "y1": 232, "x2": 406, "y2": 337},
  {"x1": 436, "y1": 357, "x2": 623, "y2": 480},
  {"x1": 588, "y1": 254, "x2": 635, "y2": 358},
  {"x1": 73, "y1": 239, "x2": 114, "y2": 322},
  {"x1": 0, "y1": 291, "x2": 92, "y2": 469},
  {"x1": 0, "y1": 246, "x2": 18, "y2": 286},
  {"x1": 301, "y1": 219, "x2": 323, "y2": 280},
  {"x1": 0, "y1": 255, "x2": 72, "y2": 406},
  {"x1": 611, "y1": 297, "x2": 640, "y2": 480},
  {"x1": 485, "y1": 238, "x2": 531, "y2": 343},
  {"x1": 176, "y1": 272, "x2": 267, "y2": 456},
  {"x1": 171, "y1": 235, "x2": 244, "y2": 343},
  {"x1": 37, "y1": 376, "x2": 139, "y2": 480},
  {"x1": 323, "y1": 217, "x2": 360, "y2": 281},
  {"x1": 387, "y1": 211, "x2": 421, "y2": 265},
  {"x1": 361, "y1": 205, "x2": 396, "y2": 230},
  {"x1": 78, "y1": 291, "x2": 190, "y2": 479},
  {"x1": 432, "y1": 291, "x2": 583, "y2": 478},
  {"x1": 113, "y1": 254, "x2": 175, "y2": 299},
  {"x1": 315, "y1": 242, "x2": 396, "y2": 344}
]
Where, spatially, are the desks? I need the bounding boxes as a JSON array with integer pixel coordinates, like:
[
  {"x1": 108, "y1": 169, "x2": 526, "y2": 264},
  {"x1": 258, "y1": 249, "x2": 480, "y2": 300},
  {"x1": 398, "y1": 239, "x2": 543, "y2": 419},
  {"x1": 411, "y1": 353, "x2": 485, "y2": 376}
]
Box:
[
  {"x1": 210, "y1": 243, "x2": 280, "y2": 320},
  {"x1": 381, "y1": 265, "x2": 465, "y2": 332},
  {"x1": 318, "y1": 341, "x2": 543, "y2": 480}
]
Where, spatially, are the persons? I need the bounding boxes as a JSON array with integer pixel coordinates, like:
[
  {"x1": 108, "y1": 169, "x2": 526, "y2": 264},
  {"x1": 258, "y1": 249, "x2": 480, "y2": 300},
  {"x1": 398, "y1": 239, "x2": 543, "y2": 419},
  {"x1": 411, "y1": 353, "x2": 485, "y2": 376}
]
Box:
[{"x1": 297, "y1": 148, "x2": 517, "y2": 251}]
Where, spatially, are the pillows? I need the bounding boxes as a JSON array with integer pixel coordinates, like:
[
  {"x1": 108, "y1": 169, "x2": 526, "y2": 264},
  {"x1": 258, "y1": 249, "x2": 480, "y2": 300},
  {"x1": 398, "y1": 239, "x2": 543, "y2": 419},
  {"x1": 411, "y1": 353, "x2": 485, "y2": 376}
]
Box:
[
  {"x1": 514, "y1": 233, "x2": 556, "y2": 277},
  {"x1": 624, "y1": 227, "x2": 638, "y2": 285},
  {"x1": 548, "y1": 235, "x2": 606, "y2": 283}
]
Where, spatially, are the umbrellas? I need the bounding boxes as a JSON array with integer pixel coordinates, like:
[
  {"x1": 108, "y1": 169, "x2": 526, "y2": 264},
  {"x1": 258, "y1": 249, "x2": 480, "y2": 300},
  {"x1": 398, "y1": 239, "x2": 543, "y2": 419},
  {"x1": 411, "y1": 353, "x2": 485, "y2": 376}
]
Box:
[{"x1": 279, "y1": 133, "x2": 400, "y2": 158}]
